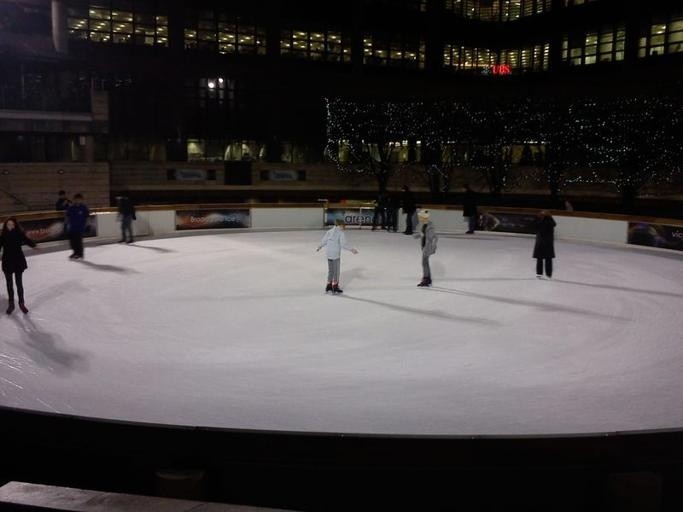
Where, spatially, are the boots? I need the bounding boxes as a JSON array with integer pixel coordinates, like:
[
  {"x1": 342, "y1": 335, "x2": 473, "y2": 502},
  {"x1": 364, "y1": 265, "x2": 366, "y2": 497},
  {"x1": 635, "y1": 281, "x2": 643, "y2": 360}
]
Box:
[
  {"x1": 331, "y1": 285, "x2": 342, "y2": 293},
  {"x1": 69, "y1": 253, "x2": 75, "y2": 258},
  {"x1": 6, "y1": 301, "x2": 14, "y2": 314},
  {"x1": 74, "y1": 250, "x2": 83, "y2": 259},
  {"x1": 325, "y1": 284, "x2": 331, "y2": 291},
  {"x1": 418, "y1": 276, "x2": 428, "y2": 286},
  {"x1": 19, "y1": 299, "x2": 28, "y2": 313}
]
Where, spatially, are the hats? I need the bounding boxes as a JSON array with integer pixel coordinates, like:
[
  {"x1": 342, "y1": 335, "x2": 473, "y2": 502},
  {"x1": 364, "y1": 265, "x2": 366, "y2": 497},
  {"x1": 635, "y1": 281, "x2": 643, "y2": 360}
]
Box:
[{"x1": 418, "y1": 209, "x2": 429, "y2": 219}]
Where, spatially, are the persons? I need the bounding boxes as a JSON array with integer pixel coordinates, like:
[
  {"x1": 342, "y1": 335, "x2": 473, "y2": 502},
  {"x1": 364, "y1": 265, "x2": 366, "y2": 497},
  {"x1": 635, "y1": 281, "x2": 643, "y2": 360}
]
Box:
[
  {"x1": 461, "y1": 182, "x2": 477, "y2": 235},
  {"x1": 66, "y1": 193, "x2": 89, "y2": 259},
  {"x1": 563, "y1": 200, "x2": 574, "y2": 211},
  {"x1": 54, "y1": 190, "x2": 74, "y2": 211},
  {"x1": 526, "y1": 210, "x2": 555, "y2": 279},
  {"x1": 315, "y1": 217, "x2": 357, "y2": 293},
  {"x1": 115, "y1": 192, "x2": 137, "y2": 244},
  {"x1": 411, "y1": 210, "x2": 437, "y2": 287},
  {"x1": 0, "y1": 216, "x2": 37, "y2": 314},
  {"x1": 370, "y1": 184, "x2": 417, "y2": 235}
]
